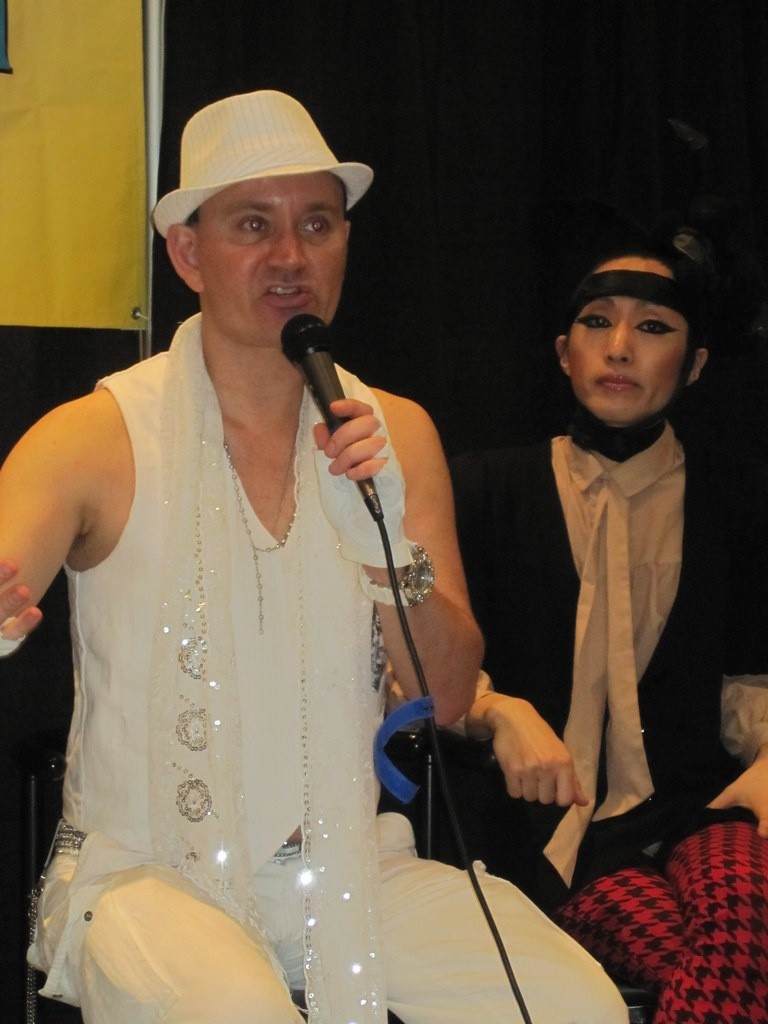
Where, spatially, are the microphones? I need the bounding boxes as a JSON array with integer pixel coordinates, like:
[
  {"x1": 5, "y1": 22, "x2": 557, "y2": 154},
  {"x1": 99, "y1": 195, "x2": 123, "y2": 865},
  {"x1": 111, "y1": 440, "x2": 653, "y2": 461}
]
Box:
[{"x1": 279, "y1": 314, "x2": 384, "y2": 522}]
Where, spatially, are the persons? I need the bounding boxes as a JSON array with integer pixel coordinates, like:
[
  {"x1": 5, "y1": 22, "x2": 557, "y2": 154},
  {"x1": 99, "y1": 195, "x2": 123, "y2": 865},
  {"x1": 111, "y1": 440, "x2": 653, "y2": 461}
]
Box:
[
  {"x1": 377, "y1": 244, "x2": 768, "y2": 1024},
  {"x1": 0, "y1": 89, "x2": 634, "y2": 1024}
]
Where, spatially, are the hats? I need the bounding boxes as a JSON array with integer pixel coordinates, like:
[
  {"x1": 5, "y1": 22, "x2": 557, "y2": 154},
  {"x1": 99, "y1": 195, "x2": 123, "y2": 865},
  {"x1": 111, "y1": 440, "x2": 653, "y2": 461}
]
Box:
[{"x1": 153, "y1": 89, "x2": 375, "y2": 248}]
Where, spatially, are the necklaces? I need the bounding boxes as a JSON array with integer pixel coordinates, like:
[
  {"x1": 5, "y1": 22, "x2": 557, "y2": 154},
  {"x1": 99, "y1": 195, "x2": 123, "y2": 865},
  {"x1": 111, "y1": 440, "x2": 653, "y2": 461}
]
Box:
[{"x1": 223, "y1": 441, "x2": 299, "y2": 633}]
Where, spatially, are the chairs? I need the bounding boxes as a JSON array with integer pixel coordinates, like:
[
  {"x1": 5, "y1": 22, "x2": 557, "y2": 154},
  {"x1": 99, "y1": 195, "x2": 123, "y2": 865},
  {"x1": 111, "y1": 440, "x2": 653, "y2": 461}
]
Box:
[
  {"x1": 405, "y1": 731, "x2": 656, "y2": 1024},
  {"x1": 9, "y1": 727, "x2": 72, "y2": 1024}
]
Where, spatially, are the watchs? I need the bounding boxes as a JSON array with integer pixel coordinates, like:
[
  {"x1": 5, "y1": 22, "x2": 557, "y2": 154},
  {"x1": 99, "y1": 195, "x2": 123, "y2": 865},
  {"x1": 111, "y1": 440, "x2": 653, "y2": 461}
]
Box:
[{"x1": 361, "y1": 542, "x2": 434, "y2": 608}]
